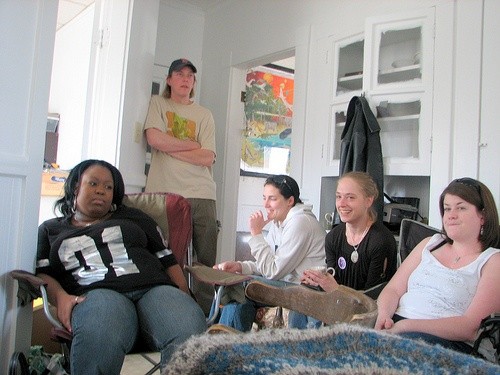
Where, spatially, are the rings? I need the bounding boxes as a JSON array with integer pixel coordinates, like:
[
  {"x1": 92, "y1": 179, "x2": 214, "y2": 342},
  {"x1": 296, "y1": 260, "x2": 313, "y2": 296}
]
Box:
[{"x1": 75, "y1": 296, "x2": 78, "y2": 302}]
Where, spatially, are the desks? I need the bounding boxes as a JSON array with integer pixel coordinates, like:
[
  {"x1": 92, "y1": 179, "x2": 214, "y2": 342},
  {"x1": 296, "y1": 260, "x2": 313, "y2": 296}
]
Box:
[{"x1": 38, "y1": 169, "x2": 69, "y2": 227}]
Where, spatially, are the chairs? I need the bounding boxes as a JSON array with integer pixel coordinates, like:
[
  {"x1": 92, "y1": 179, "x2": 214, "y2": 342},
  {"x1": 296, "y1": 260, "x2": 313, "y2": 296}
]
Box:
[
  {"x1": 205, "y1": 281, "x2": 378, "y2": 335},
  {"x1": 9, "y1": 191, "x2": 253, "y2": 375},
  {"x1": 331, "y1": 220, "x2": 401, "y2": 301},
  {"x1": 398, "y1": 218, "x2": 500, "y2": 364}
]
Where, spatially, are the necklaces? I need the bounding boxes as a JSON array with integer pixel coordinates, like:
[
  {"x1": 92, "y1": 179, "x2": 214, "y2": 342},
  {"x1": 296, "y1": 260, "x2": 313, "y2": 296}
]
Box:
[
  {"x1": 450, "y1": 242, "x2": 482, "y2": 262},
  {"x1": 74, "y1": 211, "x2": 103, "y2": 226},
  {"x1": 346, "y1": 218, "x2": 371, "y2": 263}
]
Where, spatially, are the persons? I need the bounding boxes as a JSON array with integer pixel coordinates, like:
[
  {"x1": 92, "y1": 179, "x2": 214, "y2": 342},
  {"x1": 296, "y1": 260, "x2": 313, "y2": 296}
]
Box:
[
  {"x1": 374, "y1": 177, "x2": 500, "y2": 366},
  {"x1": 143, "y1": 59, "x2": 217, "y2": 318},
  {"x1": 287, "y1": 172, "x2": 397, "y2": 329},
  {"x1": 212, "y1": 175, "x2": 328, "y2": 333},
  {"x1": 35, "y1": 159, "x2": 208, "y2": 375}
]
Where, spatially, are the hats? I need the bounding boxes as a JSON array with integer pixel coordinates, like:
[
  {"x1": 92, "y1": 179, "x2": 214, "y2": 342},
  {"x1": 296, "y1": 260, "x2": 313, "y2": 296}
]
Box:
[{"x1": 170, "y1": 59, "x2": 197, "y2": 74}]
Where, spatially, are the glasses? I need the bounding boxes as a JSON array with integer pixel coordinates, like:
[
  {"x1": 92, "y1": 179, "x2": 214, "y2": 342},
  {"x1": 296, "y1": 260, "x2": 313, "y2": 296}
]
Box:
[{"x1": 266, "y1": 177, "x2": 293, "y2": 193}]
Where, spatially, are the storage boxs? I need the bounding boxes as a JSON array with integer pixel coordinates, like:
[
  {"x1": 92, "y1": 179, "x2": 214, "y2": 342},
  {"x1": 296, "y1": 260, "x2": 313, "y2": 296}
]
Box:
[{"x1": 383, "y1": 207, "x2": 413, "y2": 222}]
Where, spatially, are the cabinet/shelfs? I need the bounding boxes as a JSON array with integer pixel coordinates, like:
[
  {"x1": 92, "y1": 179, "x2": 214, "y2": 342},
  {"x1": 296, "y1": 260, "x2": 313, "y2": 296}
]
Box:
[{"x1": 317, "y1": 6, "x2": 436, "y2": 246}]
